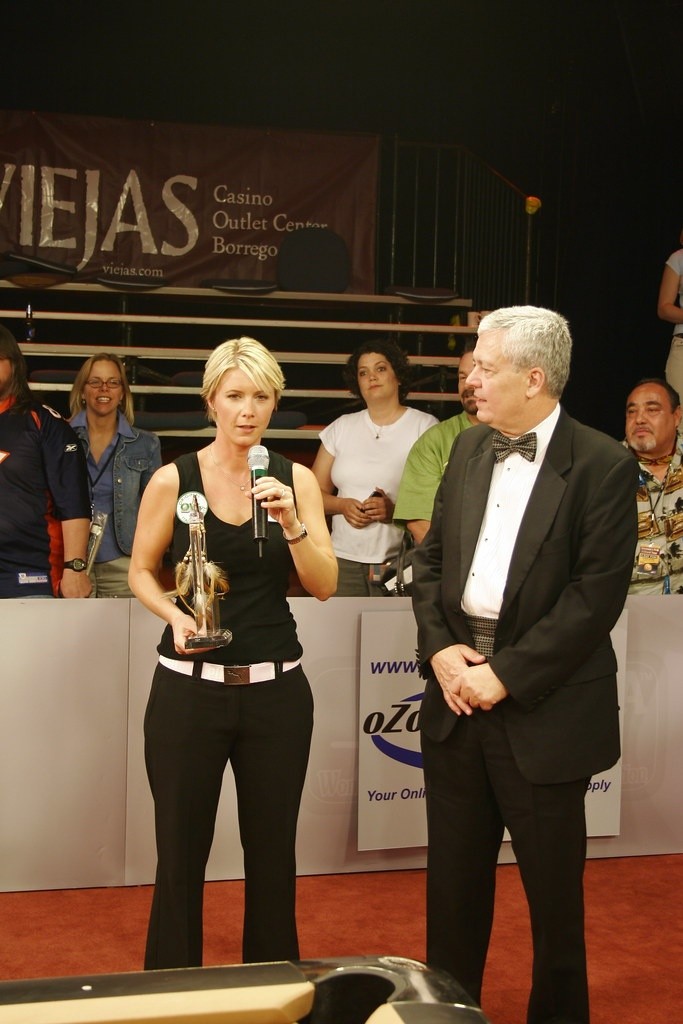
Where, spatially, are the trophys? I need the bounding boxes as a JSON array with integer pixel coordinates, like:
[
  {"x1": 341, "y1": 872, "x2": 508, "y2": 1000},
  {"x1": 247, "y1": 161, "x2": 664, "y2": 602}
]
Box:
[
  {"x1": 85, "y1": 510, "x2": 107, "y2": 576},
  {"x1": 184, "y1": 493, "x2": 233, "y2": 651}
]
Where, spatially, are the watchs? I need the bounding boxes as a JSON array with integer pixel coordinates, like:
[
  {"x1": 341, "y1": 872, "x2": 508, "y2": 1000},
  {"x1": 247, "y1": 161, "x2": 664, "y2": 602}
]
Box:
[{"x1": 63, "y1": 558, "x2": 88, "y2": 573}]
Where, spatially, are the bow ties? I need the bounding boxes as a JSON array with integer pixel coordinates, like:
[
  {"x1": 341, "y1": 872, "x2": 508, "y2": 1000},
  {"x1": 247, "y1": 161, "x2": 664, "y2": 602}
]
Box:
[{"x1": 492, "y1": 431, "x2": 536, "y2": 464}]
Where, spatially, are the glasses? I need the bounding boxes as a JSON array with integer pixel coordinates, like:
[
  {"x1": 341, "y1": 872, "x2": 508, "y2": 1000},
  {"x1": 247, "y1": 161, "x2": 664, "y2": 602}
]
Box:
[{"x1": 85, "y1": 377, "x2": 123, "y2": 388}]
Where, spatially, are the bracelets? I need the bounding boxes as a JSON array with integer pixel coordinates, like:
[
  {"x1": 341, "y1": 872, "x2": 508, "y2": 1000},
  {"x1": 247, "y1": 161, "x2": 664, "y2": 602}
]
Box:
[{"x1": 282, "y1": 522, "x2": 308, "y2": 545}]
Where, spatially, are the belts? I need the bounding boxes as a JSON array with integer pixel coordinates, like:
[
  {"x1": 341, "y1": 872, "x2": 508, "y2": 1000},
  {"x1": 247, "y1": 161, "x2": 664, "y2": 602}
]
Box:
[{"x1": 158, "y1": 654, "x2": 302, "y2": 686}]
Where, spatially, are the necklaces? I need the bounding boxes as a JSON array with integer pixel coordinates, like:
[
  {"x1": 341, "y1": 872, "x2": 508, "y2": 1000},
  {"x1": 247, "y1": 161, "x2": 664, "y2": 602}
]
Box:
[
  {"x1": 368, "y1": 405, "x2": 398, "y2": 440},
  {"x1": 209, "y1": 441, "x2": 251, "y2": 491}
]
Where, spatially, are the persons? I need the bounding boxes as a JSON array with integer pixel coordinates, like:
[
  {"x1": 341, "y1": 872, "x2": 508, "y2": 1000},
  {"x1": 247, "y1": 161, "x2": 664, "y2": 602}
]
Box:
[
  {"x1": 127, "y1": 335, "x2": 339, "y2": 972},
  {"x1": 311, "y1": 342, "x2": 443, "y2": 601},
  {"x1": 408, "y1": 305, "x2": 640, "y2": 1023},
  {"x1": 0, "y1": 324, "x2": 94, "y2": 601},
  {"x1": 395, "y1": 346, "x2": 475, "y2": 546},
  {"x1": 70, "y1": 352, "x2": 162, "y2": 600},
  {"x1": 655, "y1": 246, "x2": 683, "y2": 395},
  {"x1": 615, "y1": 377, "x2": 683, "y2": 594}
]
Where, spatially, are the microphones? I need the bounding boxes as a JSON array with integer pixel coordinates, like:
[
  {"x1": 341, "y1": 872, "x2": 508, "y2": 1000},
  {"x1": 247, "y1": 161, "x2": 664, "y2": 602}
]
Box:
[{"x1": 247, "y1": 445, "x2": 270, "y2": 558}]
